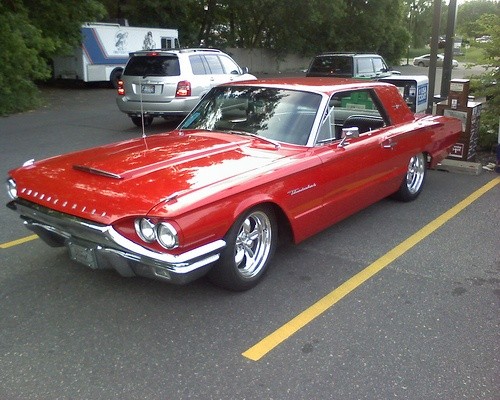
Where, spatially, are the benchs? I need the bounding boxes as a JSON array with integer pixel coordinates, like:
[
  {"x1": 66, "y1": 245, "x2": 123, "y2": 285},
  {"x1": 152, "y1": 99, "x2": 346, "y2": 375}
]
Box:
[{"x1": 291, "y1": 110, "x2": 381, "y2": 144}]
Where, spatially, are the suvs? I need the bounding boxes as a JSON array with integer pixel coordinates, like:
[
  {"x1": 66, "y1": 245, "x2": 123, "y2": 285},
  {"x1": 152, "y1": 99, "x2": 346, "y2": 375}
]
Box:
[
  {"x1": 307, "y1": 50, "x2": 402, "y2": 79},
  {"x1": 115, "y1": 46, "x2": 260, "y2": 127}
]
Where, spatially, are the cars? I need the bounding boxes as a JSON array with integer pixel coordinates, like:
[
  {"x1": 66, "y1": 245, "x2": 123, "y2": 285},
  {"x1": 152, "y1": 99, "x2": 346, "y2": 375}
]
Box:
[
  {"x1": 5, "y1": 76, "x2": 462, "y2": 295},
  {"x1": 413, "y1": 53, "x2": 459, "y2": 69}
]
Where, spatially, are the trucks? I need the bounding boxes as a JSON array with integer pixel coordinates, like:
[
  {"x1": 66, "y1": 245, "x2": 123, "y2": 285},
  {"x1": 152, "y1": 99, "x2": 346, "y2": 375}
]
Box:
[{"x1": 52, "y1": 19, "x2": 182, "y2": 90}]
